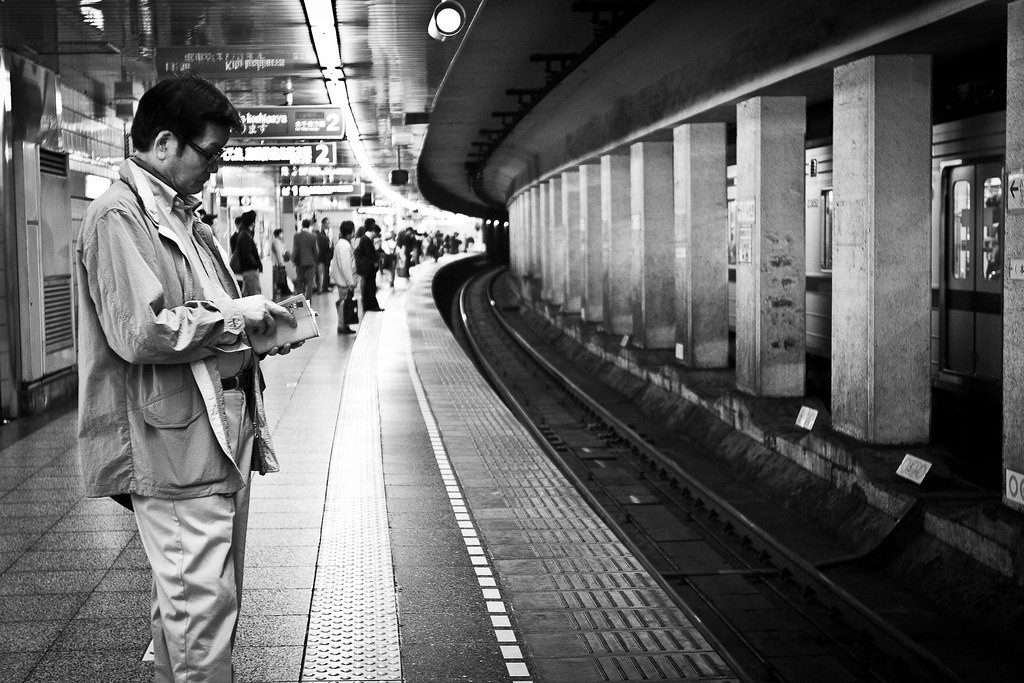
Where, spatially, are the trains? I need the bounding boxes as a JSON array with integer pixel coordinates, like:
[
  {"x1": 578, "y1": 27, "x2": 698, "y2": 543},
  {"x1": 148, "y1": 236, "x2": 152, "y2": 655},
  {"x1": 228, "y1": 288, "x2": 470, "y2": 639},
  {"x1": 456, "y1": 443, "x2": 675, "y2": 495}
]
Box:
[{"x1": 729, "y1": 109, "x2": 1005, "y2": 405}]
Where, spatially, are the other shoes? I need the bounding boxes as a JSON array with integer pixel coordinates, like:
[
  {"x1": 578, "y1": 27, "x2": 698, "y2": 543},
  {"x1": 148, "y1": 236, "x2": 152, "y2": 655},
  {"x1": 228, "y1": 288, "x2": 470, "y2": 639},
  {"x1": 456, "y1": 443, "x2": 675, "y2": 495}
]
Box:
[{"x1": 337, "y1": 326, "x2": 356, "y2": 334}]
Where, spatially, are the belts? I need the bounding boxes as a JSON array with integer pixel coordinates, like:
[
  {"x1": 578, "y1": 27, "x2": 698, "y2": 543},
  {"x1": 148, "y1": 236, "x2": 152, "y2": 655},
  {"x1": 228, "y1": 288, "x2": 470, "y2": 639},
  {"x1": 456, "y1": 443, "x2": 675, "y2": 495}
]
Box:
[{"x1": 220, "y1": 373, "x2": 244, "y2": 391}]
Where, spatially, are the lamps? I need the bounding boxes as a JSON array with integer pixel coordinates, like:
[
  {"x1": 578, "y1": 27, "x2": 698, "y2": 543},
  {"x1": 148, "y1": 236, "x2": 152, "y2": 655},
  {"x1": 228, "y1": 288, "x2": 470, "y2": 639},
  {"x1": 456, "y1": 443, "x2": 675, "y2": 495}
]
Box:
[{"x1": 427, "y1": 0, "x2": 467, "y2": 42}]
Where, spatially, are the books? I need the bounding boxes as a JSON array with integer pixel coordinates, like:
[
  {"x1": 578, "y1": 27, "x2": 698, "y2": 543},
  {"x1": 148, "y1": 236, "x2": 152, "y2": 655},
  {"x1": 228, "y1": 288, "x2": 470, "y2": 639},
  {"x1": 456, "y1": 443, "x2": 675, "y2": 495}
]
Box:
[{"x1": 248, "y1": 293, "x2": 318, "y2": 355}]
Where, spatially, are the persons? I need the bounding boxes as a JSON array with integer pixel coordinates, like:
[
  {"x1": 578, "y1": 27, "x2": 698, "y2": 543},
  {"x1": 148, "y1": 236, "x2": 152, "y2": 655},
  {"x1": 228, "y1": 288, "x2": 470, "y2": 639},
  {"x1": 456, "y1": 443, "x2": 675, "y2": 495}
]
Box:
[
  {"x1": 198, "y1": 209, "x2": 473, "y2": 335},
  {"x1": 72, "y1": 75, "x2": 303, "y2": 683}
]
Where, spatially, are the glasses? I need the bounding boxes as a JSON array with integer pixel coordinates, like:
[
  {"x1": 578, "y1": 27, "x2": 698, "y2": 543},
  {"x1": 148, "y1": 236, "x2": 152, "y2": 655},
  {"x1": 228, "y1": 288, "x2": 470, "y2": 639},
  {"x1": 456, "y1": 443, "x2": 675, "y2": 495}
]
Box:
[{"x1": 157, "y1": 126, "x2": 224, "y2": 166}]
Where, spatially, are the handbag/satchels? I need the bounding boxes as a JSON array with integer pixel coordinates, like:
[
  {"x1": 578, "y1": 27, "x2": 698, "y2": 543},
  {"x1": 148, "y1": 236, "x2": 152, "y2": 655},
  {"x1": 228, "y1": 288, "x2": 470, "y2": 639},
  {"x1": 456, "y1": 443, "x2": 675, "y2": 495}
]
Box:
[
  {"x1": 228, "y1": 249, "x2": 241, "y2": 275},
  {"x1": 342, "y1": 287, "x2": 360, "y2": 324},
  {"x1": 381, "y1": 250, "x2": 394, "y2": 268}
]
